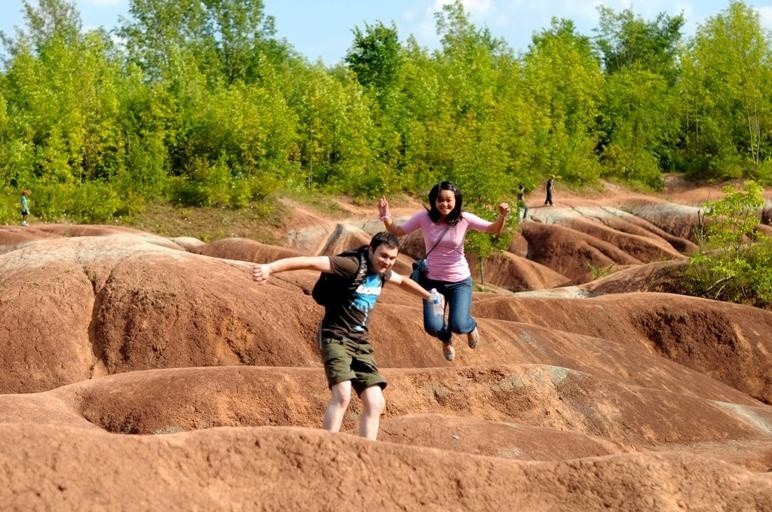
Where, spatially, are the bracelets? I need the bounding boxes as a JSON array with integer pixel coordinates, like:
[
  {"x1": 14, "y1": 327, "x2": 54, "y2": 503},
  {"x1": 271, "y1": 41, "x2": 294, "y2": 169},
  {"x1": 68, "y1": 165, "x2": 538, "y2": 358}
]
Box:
[{"x1": 499, "y1": 212, "x2": 507, "y2": 217}]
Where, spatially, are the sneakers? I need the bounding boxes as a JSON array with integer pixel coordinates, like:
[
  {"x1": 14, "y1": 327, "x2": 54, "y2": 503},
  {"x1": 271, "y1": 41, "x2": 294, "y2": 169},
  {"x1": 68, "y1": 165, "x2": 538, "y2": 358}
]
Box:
[
  {"x1": 443, "y1": 336, "x2": 455, "y2": 361},
  {"x1": 469, "y1": 326, "x2": 479, "y2": 349}
]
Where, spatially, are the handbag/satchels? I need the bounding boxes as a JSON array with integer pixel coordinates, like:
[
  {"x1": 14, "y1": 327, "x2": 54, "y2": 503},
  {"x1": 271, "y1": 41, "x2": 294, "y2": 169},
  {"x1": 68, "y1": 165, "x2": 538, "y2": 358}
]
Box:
[{"x1": 410, "y1": 259, "x2": 428, "y2": 283}]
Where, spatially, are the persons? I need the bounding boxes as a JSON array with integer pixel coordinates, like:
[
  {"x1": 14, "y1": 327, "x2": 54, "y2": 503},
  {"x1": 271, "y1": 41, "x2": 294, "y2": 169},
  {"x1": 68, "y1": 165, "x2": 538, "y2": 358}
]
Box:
[
  {"x1": 20, "y1": 190, "x2": 31, "y2": 227},
  {"x1": 378, "y1": 181, "x2": 511, "y2": 361},
  {"x1": 544, "y1": 174, "x2": 557, "y2": 207},
  {"x1": 515, "y1": 184, "x2": 529, "y2": 224},
  {"x1": 250, "y1": 229, "x2": 447, "y2": 441}
]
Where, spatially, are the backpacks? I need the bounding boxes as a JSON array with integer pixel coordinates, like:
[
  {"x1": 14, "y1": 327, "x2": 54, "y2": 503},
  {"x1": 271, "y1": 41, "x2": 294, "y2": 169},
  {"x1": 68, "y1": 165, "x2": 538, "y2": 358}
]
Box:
[{"x1": 312, "y1": 245, "x2": 386, "y2": 307}]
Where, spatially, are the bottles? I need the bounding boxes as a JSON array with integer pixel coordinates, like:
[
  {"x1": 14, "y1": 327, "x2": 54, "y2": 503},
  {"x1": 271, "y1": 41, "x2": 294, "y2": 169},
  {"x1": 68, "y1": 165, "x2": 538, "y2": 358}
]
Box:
[{"x1": 430, "y1": 288, "x2": 442, "y2": 318}]
